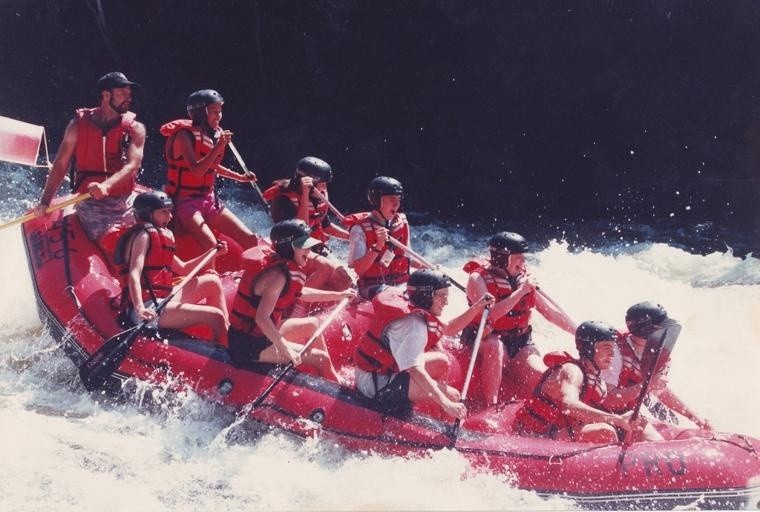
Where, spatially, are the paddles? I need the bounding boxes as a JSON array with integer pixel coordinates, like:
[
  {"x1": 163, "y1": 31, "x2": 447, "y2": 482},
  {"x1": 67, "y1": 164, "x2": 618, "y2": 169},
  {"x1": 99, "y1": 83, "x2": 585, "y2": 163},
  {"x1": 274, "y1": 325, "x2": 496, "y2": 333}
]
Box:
[
  {"x1": 208, "y1": 296, "x2": 350, "y2": 447},
  {"x1": 623, "y1": 323, "x2": 682, "y2": 445},
  {"x1": 79, "y1": 239, "x2": 223, "y2": 391}
]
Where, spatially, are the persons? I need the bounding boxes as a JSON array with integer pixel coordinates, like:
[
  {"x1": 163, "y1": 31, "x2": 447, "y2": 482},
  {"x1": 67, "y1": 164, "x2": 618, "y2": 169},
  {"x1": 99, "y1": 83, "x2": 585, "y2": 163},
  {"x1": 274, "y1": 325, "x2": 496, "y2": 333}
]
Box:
[
  {"x1": 262, "y1": 155, "x2": 353, "y2": 291},
  {"x1": 340, "y1": 176, "x2": 446, "y2": 303},
  {"x1": 160, "y1": 89, "x2": 258, "y2": 276},
  {"x1": 510, "y1": 320, "x2": 669, "y2": 444},
  {"x1": 25, "y1": 72, "x2": 147, "y2": 283},
  {"x1": 351, "y1": 268, "x2": 496, "y2": 421},
  {"x1": 460, "y1": 231, "x2": 577, "y2": 408},
  {"x1": 595, "y1": 300, "x2": 713, "y2": 441},
  {"x1": 99, "y1": 190, "x2": 230, "y2": 349},
  {"x1": 224, "y1": 218, "x2": 359, "y2": 385}
]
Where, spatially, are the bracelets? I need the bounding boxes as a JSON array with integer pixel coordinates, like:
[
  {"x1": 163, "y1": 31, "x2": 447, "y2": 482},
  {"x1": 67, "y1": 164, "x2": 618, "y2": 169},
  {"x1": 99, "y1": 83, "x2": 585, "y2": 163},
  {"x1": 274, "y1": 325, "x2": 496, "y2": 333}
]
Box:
[{"x1": 40, "y1": 197, "x2": 50, "y2": 206}]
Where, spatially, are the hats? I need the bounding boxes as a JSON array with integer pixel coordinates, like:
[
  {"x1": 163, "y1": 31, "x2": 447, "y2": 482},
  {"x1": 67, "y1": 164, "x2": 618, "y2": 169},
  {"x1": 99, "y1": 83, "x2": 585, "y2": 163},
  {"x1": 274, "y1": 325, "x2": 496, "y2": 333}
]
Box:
[
  {"x1": 98, "y1": 72, "x2": 141, "y2": 90},
  {"x1": 654, "y1": 313, "x2": 679, "y2": 327},
  {"x1": 293, "y1": 235, "x2": 323, "y2": 249}
]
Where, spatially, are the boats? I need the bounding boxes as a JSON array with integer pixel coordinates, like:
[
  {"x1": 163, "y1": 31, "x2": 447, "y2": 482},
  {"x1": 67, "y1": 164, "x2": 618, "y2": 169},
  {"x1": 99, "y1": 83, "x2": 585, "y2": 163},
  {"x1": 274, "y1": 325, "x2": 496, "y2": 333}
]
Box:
[{"x1": 13, "y1": 181, "x2": 760, "y2": 512}]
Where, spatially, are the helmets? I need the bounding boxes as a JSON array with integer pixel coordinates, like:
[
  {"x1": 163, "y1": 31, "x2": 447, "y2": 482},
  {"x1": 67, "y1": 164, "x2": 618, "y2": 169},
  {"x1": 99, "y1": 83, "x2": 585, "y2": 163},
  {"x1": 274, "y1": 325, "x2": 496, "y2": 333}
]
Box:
[
  {"x1": 186, "y1": 89, "x2": 224, "y2": 123},
  {"x1": 133, "y1": 191, "x2": 174, "y2": 223},
  {"x1": 626, "y1": 301, "x2": 668, "y2": 335},
  {"x1": 490, "y1": 231, "x2": 529, "y2": 269},
  {"x1": 407, "y1": 269, "x2": 451, "y2": 308},
  {"x1": 270, "y1": 220, "x2": 312, "y2": 259},
  {"x1": 576, "y1": 320, "x2": 618, "y2": 359},
  {"x1": 367, "y1": 177, "x2": 403, "y2": 210},
  {"x1": 295, "y1": 157, "x2": 333, "y2": 193}
]
